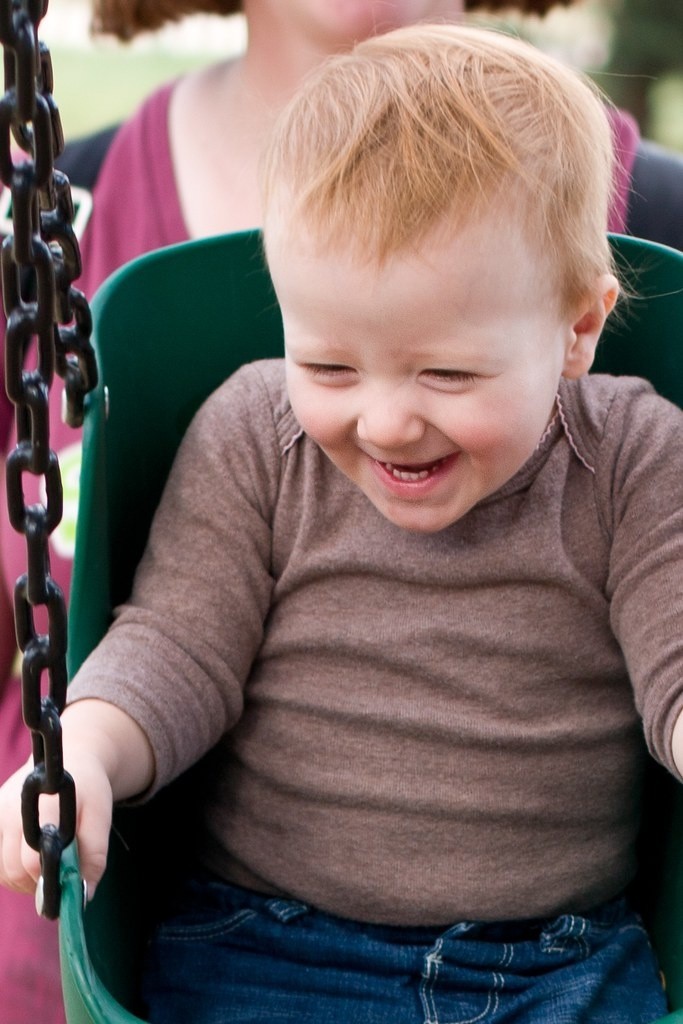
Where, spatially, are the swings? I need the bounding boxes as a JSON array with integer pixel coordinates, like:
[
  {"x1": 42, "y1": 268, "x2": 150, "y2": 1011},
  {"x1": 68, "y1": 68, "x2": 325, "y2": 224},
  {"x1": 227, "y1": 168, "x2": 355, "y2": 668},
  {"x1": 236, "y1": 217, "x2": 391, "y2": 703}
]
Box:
[{"x1": 1, "y1": 2, "x2": 683, "y2": 1023}]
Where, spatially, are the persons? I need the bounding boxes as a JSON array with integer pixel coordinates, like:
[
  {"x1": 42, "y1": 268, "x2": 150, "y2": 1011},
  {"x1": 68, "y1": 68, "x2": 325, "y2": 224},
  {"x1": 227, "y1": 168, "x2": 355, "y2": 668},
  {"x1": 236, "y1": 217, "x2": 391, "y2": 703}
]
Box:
[
  {"x1": 2, "y1": 0, "x2": 681, "y2": 1021},
  {"x1": 2, "y1": 24, "x2": 681, "y2": 1024}
]
